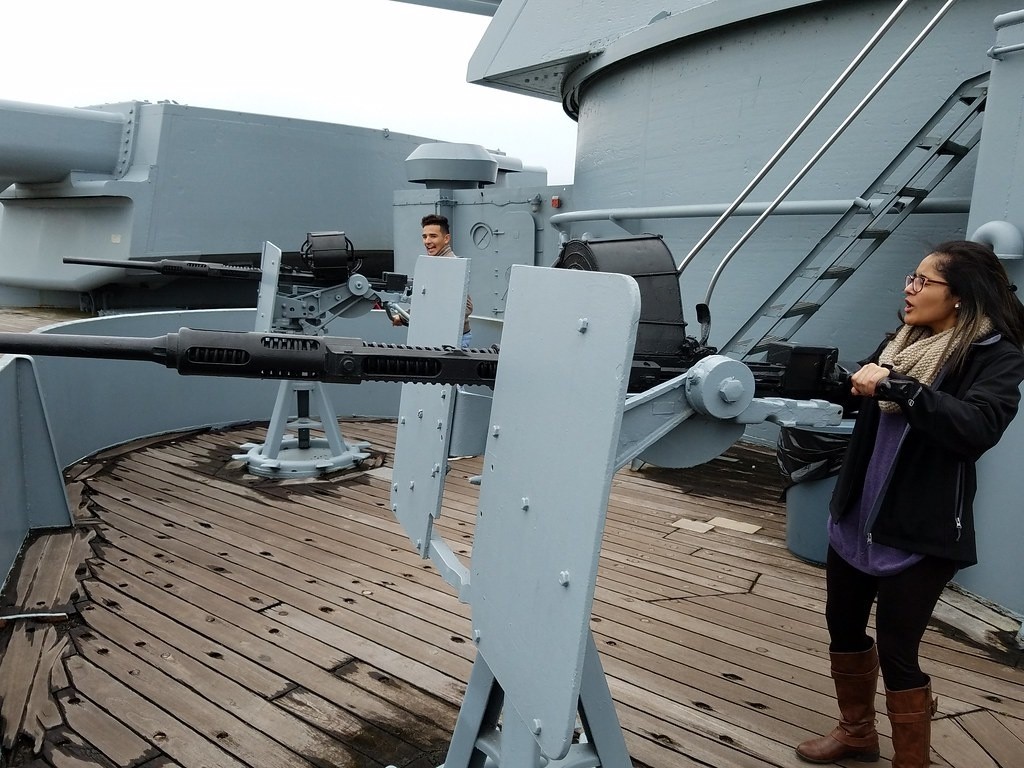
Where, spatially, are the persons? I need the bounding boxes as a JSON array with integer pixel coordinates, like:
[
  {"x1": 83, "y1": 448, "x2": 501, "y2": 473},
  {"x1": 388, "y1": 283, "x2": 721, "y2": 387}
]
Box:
[
  {"x1": 796, "y1": 241, "x2": 1023, "y2": 768},
  {"x1": 389, "y1": 215, "x2": 473, "y2": 349}
]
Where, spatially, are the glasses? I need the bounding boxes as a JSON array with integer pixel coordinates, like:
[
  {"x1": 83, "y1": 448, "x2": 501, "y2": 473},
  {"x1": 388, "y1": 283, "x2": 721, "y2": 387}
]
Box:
[{"x1": 905, "y1": 274, "x2": 950, "y2": 293}]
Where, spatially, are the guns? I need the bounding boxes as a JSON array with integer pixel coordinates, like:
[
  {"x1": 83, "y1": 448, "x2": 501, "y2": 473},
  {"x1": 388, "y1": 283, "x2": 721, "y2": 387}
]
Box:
[
  {"x1": 1, "y1": 232, "x2": 921, "y2": 418},
  {"x1": 62, "y1": 235, "x2": 409, "y2": 292}
]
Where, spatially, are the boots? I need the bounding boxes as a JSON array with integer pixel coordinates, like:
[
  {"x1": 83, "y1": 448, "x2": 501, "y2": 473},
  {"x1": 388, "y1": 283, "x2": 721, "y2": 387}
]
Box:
[
  {"x1": 796, "y1": 635, "x2": 880, "y2": 764},
  {"x1": 884, "y1": 674, "x2": 938, "y2": 768}
]
